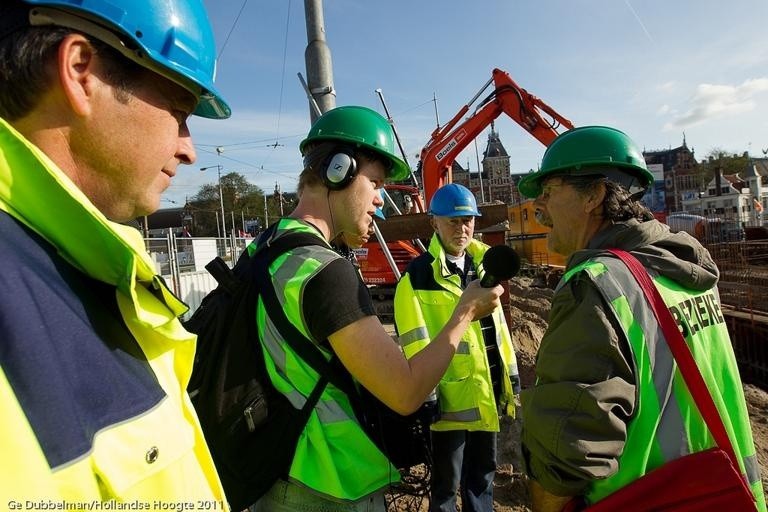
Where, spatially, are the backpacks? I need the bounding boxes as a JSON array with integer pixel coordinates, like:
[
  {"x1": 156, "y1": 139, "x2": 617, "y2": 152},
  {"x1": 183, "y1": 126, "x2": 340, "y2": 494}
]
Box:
[{"x1": 181, "y1": 218, "x2": 339, "y2": 512}]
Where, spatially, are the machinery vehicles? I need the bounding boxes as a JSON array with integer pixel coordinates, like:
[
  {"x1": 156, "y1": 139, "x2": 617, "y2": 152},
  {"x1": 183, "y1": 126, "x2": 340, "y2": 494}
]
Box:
[
  {"x1": 665, "y1": 214, "x2": 745, "y2": 245},
  {"x1": 343, "y1": 68, "x2": 580, "y2": 328}
]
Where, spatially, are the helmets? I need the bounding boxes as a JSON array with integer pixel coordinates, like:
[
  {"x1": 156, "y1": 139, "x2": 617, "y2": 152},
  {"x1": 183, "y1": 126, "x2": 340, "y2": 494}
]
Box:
[
  {"x1": 428, "y1": 183, "x2": 482, "y2": 217},
  {"x1": 27, "y1": 0, "x2": 231, "y2": 120},
  {"x1": 300, "y1": 105, "x2": 409, "y2": 181},
  {"x1": 518, "y1": 126, "x2": 654, "y2": 198}
]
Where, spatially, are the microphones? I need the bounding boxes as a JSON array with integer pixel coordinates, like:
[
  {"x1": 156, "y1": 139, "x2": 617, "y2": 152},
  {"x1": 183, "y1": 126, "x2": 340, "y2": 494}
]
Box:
[{"x1": 480, "y1": 245, "x2": 520, "y2": 289}]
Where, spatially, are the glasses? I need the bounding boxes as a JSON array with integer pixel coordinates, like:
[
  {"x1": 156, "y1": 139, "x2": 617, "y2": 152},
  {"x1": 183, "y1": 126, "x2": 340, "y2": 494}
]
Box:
[{"x1": 541, "y1": 182, "x2": 573, "y2": 197}]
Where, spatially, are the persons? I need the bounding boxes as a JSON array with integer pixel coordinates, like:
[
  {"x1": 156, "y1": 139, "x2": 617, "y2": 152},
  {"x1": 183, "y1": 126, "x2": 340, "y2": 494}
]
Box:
[
  {"x1": 0, "y1": 0, "x2": 234, "y2": 512},
  {"x1": 392, "y1": 181, "x2": 522, "y2": 512},
  {"x1": 254, "y1": 105, "x2": 504, "y2": 512},
  {"x1": 517, "y1": 125, "x2": 767, "y2": 512},
  {"x1": 330, "y1": 187, "x2": 386, "y2": 262}
]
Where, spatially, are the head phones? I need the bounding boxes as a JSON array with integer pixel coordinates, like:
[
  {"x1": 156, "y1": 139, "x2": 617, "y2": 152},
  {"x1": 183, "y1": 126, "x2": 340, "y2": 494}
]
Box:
[{"x1": 320, "y1": 149, "x2": 359, "y2": 192}]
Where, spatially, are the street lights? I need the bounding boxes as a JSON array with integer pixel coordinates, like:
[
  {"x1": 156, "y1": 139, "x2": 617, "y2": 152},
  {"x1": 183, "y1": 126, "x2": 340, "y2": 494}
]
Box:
[{"x1": 200, "y1": 164, "x2": 228, "y2": 258}]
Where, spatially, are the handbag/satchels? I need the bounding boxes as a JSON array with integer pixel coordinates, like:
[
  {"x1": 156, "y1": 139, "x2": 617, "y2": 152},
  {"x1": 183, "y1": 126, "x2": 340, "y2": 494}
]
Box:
[{"x1": 587, "y1": 445, "x2": 757, "y2": 512}]
